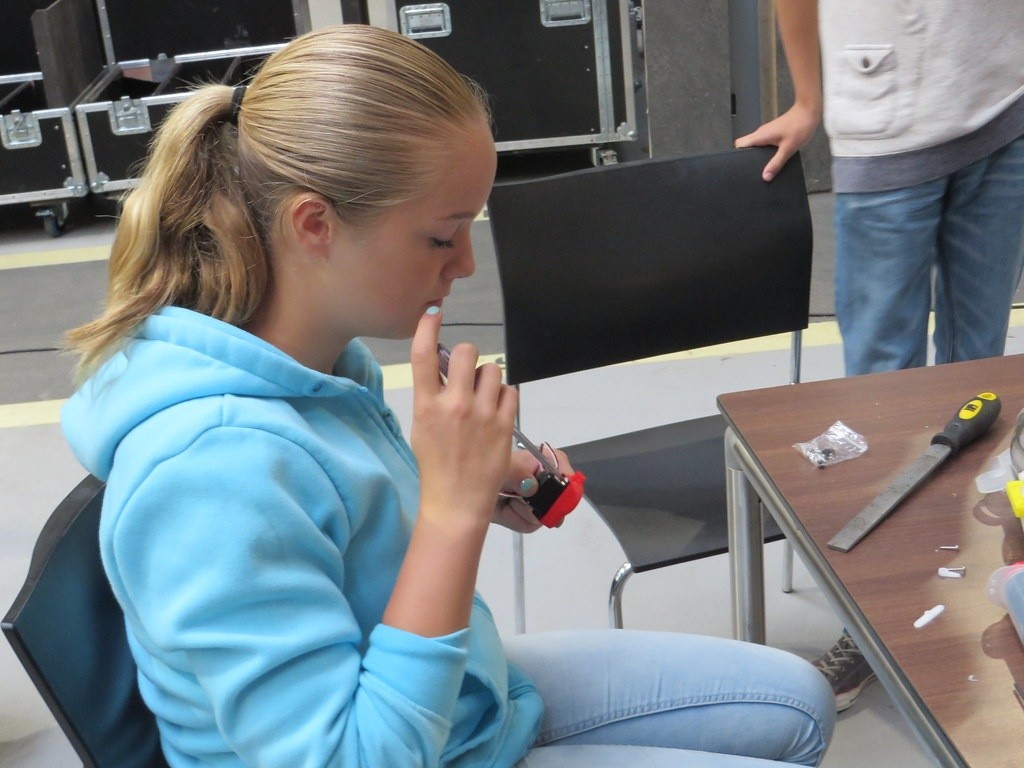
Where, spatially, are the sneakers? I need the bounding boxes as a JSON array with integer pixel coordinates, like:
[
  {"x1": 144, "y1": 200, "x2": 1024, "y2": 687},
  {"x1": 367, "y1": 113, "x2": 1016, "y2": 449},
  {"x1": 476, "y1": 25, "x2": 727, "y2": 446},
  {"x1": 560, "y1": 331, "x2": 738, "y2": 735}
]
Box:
[{"x1": 812, "y1": 626, "x2": 878, "y2": 714}]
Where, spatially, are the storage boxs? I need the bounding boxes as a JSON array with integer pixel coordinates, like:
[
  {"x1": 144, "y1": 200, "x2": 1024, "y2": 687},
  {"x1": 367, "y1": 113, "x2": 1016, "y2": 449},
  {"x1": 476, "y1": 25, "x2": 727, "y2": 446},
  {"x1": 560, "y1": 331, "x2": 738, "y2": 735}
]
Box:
[{"x1": 0, "y1": 2, "x2": 636, "y2": 234}]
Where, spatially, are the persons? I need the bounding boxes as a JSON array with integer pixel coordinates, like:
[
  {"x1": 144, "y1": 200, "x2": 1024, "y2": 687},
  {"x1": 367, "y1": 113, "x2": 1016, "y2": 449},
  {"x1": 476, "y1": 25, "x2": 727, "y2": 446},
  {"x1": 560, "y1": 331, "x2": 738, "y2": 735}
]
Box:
[
  {"x1": 732, "y1": 0, "x2": 1024, "y2": 716},
  {"x1": 53, "y1": 20, "x2": 842, "y2": 768}
]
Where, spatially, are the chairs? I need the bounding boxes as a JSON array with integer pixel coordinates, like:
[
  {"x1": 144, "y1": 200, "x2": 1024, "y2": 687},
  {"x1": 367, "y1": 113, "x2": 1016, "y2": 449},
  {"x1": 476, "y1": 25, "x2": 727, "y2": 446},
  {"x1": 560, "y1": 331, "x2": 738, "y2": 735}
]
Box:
[
  {"x1": 0, "y1": 469, "x2": 171, "y2": 768},
  {"x1": 487, "y1": 141, "x2": 817, "y2": 635}
]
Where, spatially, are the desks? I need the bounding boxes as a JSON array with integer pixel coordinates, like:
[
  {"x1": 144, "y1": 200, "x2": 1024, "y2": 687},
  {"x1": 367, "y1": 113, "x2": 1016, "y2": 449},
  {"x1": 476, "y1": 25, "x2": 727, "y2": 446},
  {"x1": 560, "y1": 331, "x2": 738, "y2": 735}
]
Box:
[{"x1": 711, "y1": 355, "x2": 1024, "y2": 768}]
